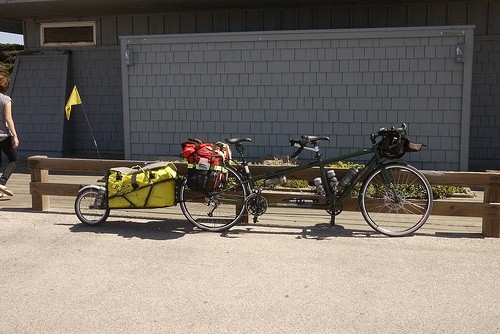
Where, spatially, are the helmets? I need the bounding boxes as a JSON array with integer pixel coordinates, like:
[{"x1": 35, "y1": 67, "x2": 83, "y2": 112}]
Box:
[{"x1": 378, "y1": 132, "x2": 409, "y2": 160}]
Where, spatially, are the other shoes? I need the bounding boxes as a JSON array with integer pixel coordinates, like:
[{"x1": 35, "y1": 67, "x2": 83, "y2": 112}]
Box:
[{"x1": 0, "y1": 184, "x2": 14, "y2": 198}]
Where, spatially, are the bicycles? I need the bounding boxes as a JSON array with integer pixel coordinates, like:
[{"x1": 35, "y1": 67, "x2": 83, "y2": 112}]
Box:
[{"x1": 177, "y1": 122, "x2": 434, "y2": 238}]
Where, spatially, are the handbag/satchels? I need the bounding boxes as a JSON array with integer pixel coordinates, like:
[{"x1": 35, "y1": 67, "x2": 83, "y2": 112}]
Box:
[
  {"x1": 179, "y1": 137, "x2": 232, "y2": 191},
  {"x1": 107, "y1": 161, "x2": 178, "y2": 209}
]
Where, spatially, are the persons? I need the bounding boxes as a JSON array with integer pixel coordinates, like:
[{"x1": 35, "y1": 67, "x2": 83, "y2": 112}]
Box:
[{"x1": 0, "y1": 71, "x2": 19, "y2": 198}]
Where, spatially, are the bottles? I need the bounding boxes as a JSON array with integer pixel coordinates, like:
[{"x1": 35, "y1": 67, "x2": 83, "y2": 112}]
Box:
[
  {"x1": 339, "y1": 166, "x2": 360, "y2": 188},
  {"x1": 314, "y1": 177, "x2": 325, "y2": 198},
  {"x1": 263, "y1": 176, "x2": 286, "y2": 187},
  {"x1": 327, "y1": 170, "x2": 339, "y2": 192}
]
